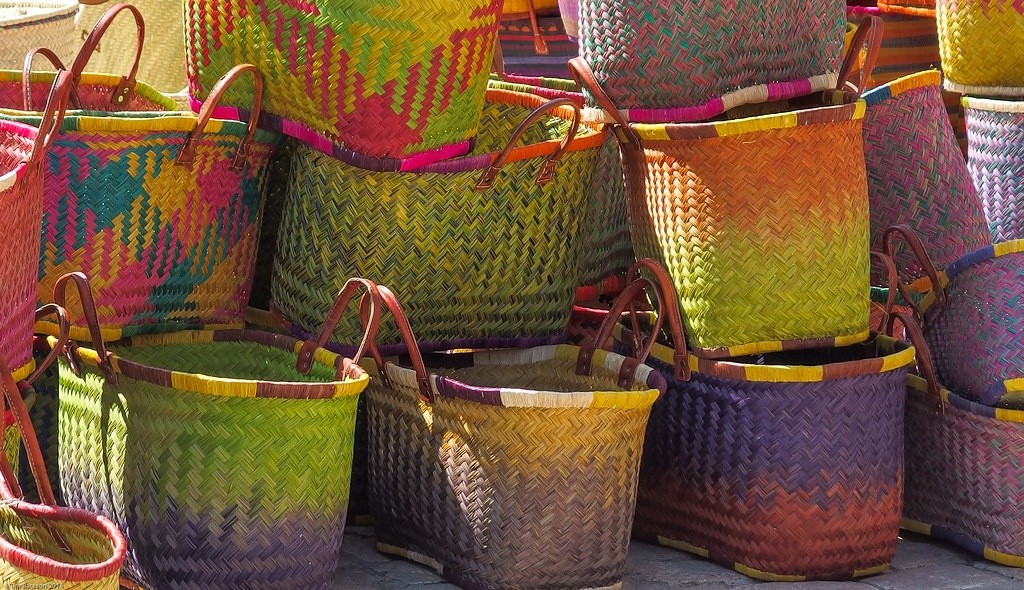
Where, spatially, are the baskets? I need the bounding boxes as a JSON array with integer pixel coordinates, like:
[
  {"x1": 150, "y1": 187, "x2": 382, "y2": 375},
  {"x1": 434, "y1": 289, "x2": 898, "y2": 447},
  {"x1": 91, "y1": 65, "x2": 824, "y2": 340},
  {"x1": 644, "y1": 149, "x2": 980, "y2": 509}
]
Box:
[{"x1": 1, "y1": 1, "x2": 1023, "y2": 590}]
